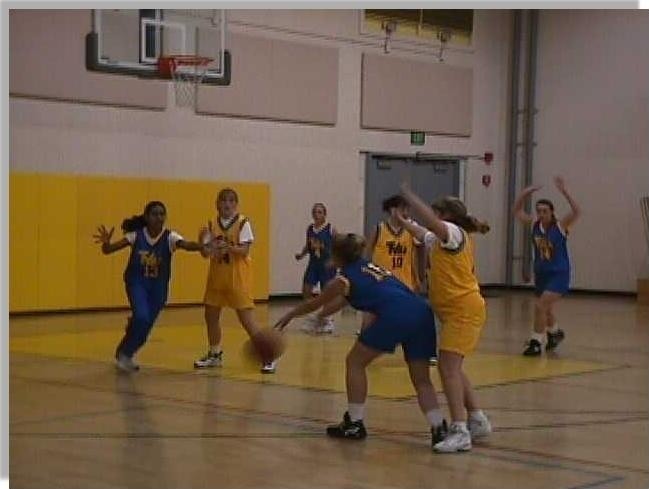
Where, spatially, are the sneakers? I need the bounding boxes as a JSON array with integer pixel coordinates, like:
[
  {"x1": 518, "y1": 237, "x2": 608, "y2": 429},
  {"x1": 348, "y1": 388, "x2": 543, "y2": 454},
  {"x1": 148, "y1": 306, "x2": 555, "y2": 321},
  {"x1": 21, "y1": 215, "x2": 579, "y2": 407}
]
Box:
[
  {"x1": 430, "y1": 418, "x2": 447, "y2": 453},
  {"x1": 192, "y1": 350, "x2": 223, "y2": 368},
  {"x1": 431, "y1": 422, "x2": 473, "y2": 454},
  {"x1": 113, "y1": 353, "x2": 141, "y2": 372},
  {"x1": 428, "y1": 352, "x2": 439, "y2": 365},
  {"x1": 300, "y1": 316, "x2": 335, "y2": 333},
  {"x1": 259, "y1": 359, "x2": 277, "y2": 374},
  {"x1": 468, "y1": 408, "x2": 492, "y2": 438},
  {"x1": 326, "y1": 411, "x2": 368, "y2": 441},
  {"x1": 522, "y1": 339, "x2": 542, "y2": 355},
  {"x1": 545, "y1": 328, "x2": 566, "y2": 352}
]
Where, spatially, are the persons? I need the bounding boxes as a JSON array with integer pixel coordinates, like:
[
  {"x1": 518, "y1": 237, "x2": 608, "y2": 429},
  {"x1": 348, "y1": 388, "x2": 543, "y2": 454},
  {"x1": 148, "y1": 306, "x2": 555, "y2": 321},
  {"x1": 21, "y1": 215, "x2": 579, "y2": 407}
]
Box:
[
  {"x1": 392, "y1": 174, "x2": 493, "y2": 453},
  {"x1": 359, "y1": 193, "x2": 427, "y2": 296},
  {"x1": 271, "y1": 231, "x2": 450, "y2": 452},
  {"x1": 193, "y1": 187, "x2": 283, "y2": 374},
  {"x1": 511, "y1": 174, "x2": 584, "y2": 358},
  {"x1": 92, "y1": 199, "x2": 207, "y2": 374},
  {"x1": 294, "y1": 202, "x2": 341, "y2": 333}
]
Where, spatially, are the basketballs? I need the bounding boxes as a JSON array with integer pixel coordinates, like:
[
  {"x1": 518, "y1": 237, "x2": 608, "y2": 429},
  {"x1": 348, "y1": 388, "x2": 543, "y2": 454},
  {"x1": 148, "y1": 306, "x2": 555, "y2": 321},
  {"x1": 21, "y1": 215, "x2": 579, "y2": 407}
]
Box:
[{"x1": 247, "y1": 326, "x2": 287, "y2": 362}]
